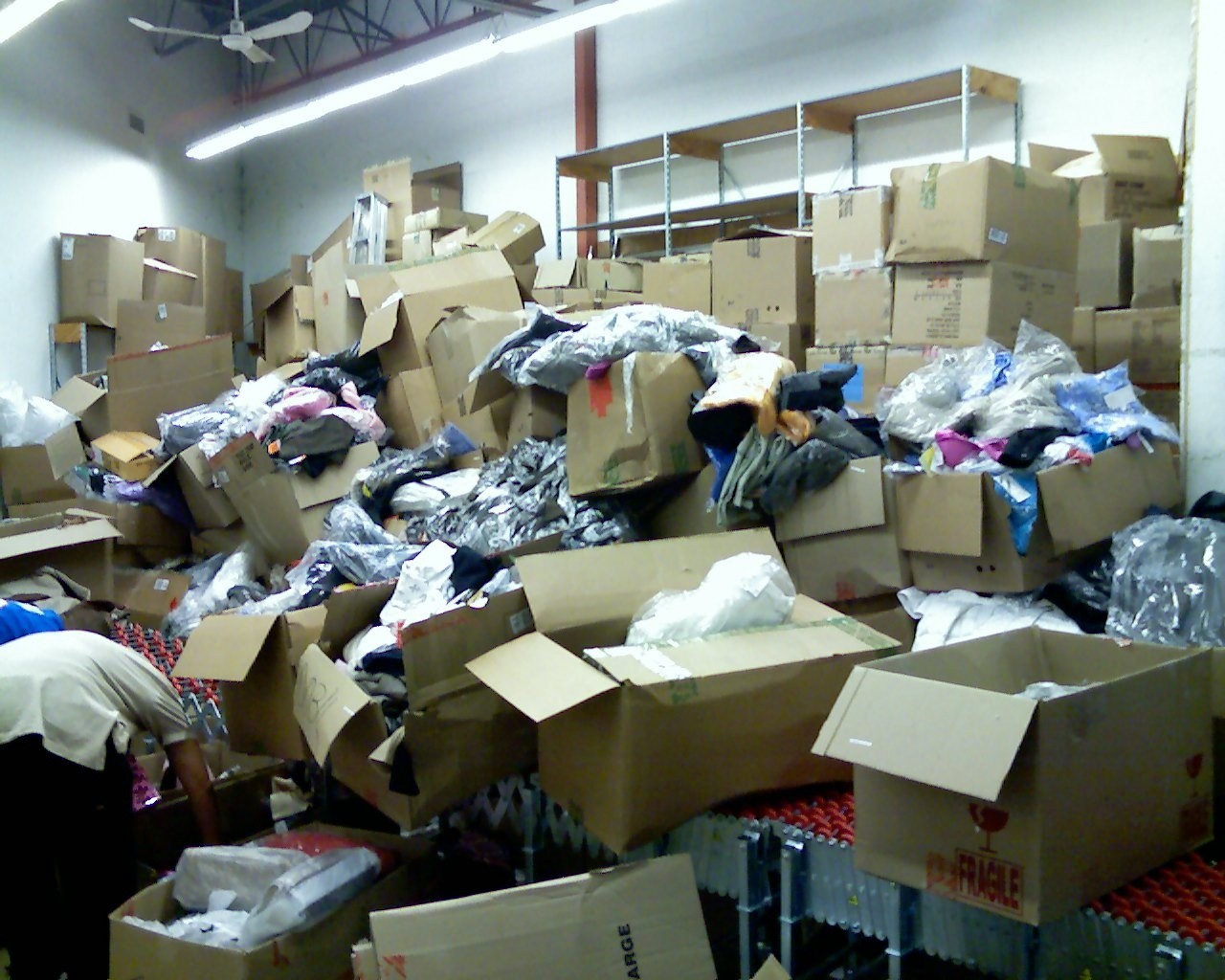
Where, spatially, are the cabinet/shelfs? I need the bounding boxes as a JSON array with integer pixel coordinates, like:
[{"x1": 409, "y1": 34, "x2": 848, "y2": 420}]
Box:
[{"x1": 556, "y1": 65, "x2": 1023, "y2": 259}]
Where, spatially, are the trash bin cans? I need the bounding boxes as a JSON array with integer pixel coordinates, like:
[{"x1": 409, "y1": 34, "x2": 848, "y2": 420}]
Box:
[{"x1": 136, "y1": 745, "x2": 293, "y2": 875}]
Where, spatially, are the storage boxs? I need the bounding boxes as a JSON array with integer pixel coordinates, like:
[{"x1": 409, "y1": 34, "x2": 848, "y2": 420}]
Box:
[{"x1": 0, "y1": 135, "x2": 1225, "y2": 980}]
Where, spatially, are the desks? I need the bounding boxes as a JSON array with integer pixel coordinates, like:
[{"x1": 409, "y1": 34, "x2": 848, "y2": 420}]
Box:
[{"x1": 513, "y1": 780, "x2": 1225, "y2": 980}]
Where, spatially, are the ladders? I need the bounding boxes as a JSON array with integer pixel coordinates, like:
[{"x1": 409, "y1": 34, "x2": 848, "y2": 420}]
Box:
[{"x1": 350, "y1": 191, "x2": 388, "y2": 265}]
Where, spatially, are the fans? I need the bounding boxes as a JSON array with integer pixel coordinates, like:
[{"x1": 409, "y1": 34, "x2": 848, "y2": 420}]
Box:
[{"x1": 127, "y1": 0, "x2": 313, "y2": 63}]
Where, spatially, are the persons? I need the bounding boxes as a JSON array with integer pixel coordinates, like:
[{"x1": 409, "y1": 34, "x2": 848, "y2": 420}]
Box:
[{"x1": 0, "y1": 627, "x2": 216, "y2": 980}]
